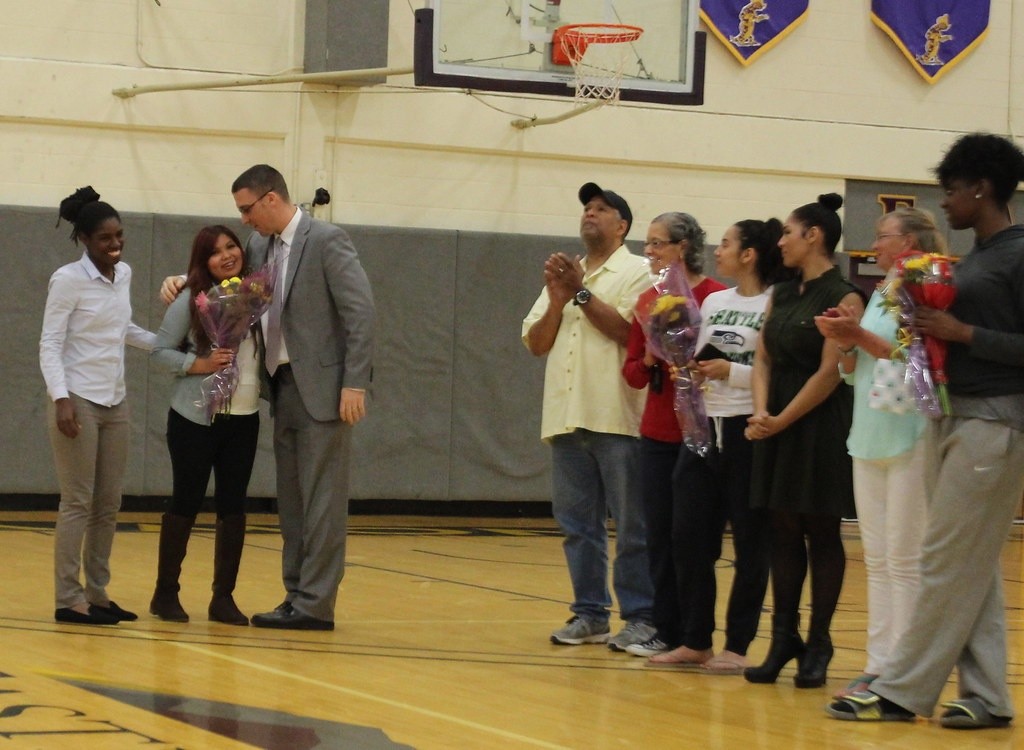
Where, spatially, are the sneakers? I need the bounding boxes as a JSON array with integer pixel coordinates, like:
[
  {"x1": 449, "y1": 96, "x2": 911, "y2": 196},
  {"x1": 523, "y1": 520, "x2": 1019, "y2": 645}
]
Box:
[
  {"x1": 551, "y1": 615, "x2": 611, "y2": 645},
  {"x1": 625, "y1": 634, "x2": 669, "y2": 655},
  {"x1": 606, "y1": 624, "x2": 656, "y2": 653}
]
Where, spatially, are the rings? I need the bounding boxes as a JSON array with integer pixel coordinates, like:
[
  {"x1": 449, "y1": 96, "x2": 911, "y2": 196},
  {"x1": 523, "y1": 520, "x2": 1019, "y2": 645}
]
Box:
[{"x1": 559, "y1": 268, "x2": 563, "y2": 272}]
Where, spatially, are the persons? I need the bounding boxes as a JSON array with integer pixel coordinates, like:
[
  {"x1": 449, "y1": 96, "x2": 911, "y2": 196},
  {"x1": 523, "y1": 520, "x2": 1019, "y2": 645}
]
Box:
[
  {"x1": 623, "y1": 213, "x2": 728, "y2": 654},
  {"x1": 827, "y1": 133, "x2": 1024, "y2": 729},
  {"x1": 744, "y1": 193, "x2": 865, "y2": 687},
  {"x1": 40, "y1": 185, "x2": 158, "y2": 626},
  {"x1": 158, "y1": 164, "x2": 376, "y2": 631},
  {"x1": 521, "y1": 182, "x2": 657, "y2": 655},
  {"x1": 645, "y1": 219, "x2": 785, "y2": 671},
  {"x1": 149, "y1": 225, "x2": 259, "y2": 625},
  {"x1": 814, "y1": 208, "x2": 948, "y2": 701}
]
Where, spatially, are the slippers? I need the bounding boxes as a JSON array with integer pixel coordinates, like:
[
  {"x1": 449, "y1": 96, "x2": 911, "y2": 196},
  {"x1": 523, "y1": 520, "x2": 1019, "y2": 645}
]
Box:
[
  {"x1": 826, "y1": 691, "x2": 915, "y2": 720},
  {"x1": 700, "y1": 656, "x2": 746, "y2": 675},
  {"x1": 643, "y1": 646, "x2": 703, "y2": 668},
  {"x1": 841, "y1": 675, "x2": 879, "y2": 696},
  {"x1": 939, "y1": 699, "x2": 1010, "y2": 728}
]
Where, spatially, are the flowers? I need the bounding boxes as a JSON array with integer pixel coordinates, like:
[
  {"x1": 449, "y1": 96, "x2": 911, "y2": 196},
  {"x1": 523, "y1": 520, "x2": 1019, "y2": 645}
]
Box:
[
  {"x1": 648, "y1": 294, "x2": 711, "y2": 431},
  {"x1": 194, "y1": 275, "x2": 274, "y2": 426},
  {"x1": 874, "y1": 251, "x2": 960, "y2": 420}
]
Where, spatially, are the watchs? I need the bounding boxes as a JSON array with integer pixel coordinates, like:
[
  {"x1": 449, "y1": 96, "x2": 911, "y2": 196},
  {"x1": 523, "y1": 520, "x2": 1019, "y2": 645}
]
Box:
[{"x1": 574, "y1": 290, "x2": 591, "y2": 305}]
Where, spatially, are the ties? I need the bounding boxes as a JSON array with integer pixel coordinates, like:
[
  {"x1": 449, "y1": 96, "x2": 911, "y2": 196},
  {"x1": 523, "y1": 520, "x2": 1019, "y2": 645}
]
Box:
[{"x1": 265, "y1": 237, "x2": 285, "y2": 377}]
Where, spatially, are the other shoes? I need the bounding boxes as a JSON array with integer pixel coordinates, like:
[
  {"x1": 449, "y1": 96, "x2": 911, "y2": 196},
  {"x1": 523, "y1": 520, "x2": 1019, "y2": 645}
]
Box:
[
  {"x1": 55, "y1": 604, "x2": 120, "y2": 625},
  {"x1": 87, "y1": 600, "x2": 138, "y2": 621}
]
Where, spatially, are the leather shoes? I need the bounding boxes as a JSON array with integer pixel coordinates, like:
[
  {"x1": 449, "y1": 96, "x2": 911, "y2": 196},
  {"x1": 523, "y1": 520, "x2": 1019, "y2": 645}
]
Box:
[{"x1": 250, "y1": 600, "x2": 334, "y2": 630}]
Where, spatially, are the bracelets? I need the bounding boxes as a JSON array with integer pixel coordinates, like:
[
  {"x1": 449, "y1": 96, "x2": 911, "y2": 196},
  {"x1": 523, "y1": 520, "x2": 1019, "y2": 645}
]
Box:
[{"x1": 838, "y1": 344, "x2": 856, "y2": 355}]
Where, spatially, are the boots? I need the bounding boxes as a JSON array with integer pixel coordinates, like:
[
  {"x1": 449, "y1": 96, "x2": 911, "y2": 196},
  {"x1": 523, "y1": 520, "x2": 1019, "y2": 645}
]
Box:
[
  {"x1": 794, "y1": 615, "x2": 833, "y2": 688},
  {"x1": 150, "y1": 515, "x2": 196, "y2": 623},
  {"x1": 208, "y1": 515, "x2": 248, "y2": 626},
  {"x1": 743, "y1": 613, "x2": 804, "y2": 684}
]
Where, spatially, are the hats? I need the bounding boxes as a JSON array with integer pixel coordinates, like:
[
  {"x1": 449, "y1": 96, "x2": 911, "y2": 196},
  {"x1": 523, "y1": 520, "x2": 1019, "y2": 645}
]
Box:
[{"x1": 578, "y1": 182, "x2": 633, "y2": 240}]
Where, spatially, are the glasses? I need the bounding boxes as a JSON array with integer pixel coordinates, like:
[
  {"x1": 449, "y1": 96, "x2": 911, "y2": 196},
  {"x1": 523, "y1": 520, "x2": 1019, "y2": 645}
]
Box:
[
  {"x1": 645, "y1": 237, "x2": 683, "y2": 248},
  {"x1": 239, "y1": 186, "x2": 275, "y2": 215},
  {"x1": 876, "y1": 232, "x2": 909, "y2": 241}
]
